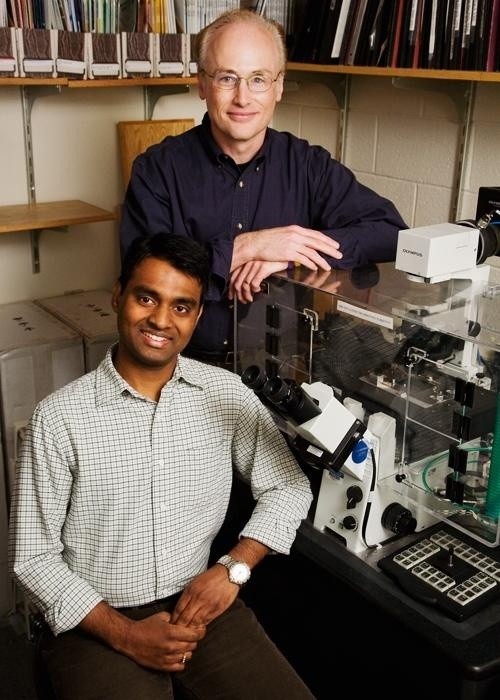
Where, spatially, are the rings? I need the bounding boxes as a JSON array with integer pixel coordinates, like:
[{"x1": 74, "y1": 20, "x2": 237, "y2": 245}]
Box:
[{"x1": 181, "y1": 653, "x2": 186, "y2": 664}]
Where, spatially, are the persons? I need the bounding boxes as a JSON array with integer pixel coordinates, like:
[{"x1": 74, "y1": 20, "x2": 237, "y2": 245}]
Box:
[
  {"x1": 4, "y1": 230, "x2": 324, "y2": 700},
  {"x1": 117, "y1": 10, "x2": 410, "y2": 378}
]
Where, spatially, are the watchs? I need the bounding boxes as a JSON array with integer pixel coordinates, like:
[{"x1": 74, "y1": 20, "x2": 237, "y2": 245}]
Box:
[{"x1": 217, "y1": 554, "x2": 253, "y2": 585}]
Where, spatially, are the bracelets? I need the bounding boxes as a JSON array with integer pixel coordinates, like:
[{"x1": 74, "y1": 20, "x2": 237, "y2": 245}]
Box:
[
  {"x1": 295, "y1": 262, "x2": 301, "y2": 267},
  {"x1": 287, "y1": 261, "x2": 295, "y2": 269}
]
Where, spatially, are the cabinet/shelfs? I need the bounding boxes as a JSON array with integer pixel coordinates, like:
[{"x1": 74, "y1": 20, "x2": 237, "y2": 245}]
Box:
[{"x1": 0, "y1": 77, "x2": 117, "y2": 272}]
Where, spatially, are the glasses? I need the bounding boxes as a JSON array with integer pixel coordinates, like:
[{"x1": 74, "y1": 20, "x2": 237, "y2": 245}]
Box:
[{"x1": 197, "y1": 68, "x2": 284, "y2": 92}]
